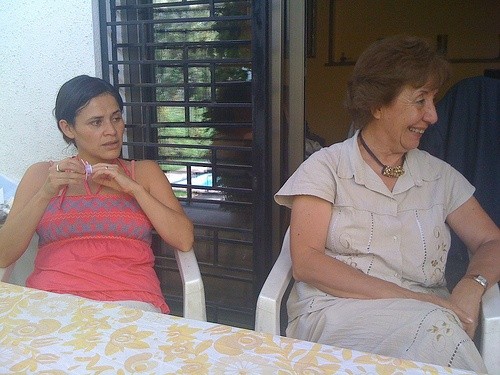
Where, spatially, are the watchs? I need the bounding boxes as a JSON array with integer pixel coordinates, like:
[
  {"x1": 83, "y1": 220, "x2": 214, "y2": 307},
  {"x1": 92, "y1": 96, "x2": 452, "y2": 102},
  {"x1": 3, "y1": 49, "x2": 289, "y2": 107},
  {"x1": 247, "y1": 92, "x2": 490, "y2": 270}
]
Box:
[{"x1": 462, "y1": 274, "x2": 489, "y2": 296}]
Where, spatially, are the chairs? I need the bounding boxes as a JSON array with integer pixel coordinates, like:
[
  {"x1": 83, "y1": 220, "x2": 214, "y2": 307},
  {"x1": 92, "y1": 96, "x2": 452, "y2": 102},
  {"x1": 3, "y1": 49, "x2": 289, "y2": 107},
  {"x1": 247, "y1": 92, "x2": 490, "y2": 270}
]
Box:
[
  {"x1": 254, "y1": 224, "x2": 500, "y2": 374},
  {"x1": 0, "y1": 247, "x2": 207, "y2": 322}
]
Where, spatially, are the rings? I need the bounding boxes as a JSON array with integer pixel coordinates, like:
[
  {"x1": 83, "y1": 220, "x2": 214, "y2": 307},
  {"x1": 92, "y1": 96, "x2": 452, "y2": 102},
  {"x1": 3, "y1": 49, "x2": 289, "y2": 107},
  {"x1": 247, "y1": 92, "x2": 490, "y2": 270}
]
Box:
[
  {"x1": 105, "y1": 165, "x2": 108, "y2": 170},
  {"x1": 56, "y1": 164, "x2": 59, "y2": 172}
]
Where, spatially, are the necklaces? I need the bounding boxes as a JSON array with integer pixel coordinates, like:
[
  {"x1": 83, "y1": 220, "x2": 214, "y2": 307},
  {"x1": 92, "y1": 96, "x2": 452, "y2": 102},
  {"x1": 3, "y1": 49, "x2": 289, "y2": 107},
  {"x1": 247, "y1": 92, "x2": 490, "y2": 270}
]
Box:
[{"x1": 359, "y1": 128, "x2": 405, "y2": 178}]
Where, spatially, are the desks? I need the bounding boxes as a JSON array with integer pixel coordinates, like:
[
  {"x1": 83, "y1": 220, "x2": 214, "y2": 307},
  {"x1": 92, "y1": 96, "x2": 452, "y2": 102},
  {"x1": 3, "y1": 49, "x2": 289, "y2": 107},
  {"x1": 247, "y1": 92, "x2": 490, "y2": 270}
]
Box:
[{"x1": 0, "y1": 279, "x2": 482, "y2": 374}]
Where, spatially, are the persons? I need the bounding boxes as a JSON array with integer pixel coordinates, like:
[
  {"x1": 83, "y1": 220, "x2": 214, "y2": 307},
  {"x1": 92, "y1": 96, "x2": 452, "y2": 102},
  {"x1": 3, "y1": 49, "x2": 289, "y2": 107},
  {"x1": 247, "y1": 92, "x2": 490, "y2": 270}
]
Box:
[
  {"x1": 275, "y1": 32, "x2": 500, "y2": 375},
  {"x1": 0, "y1": 76, "x2": 194, "y2": 314}
]
